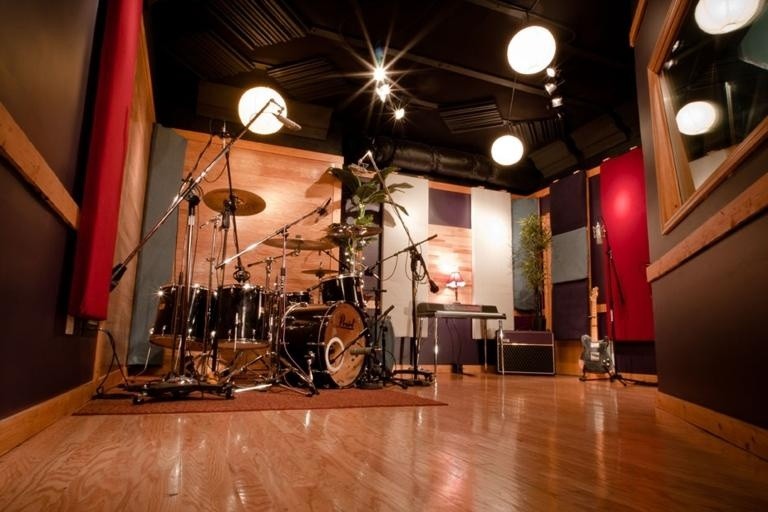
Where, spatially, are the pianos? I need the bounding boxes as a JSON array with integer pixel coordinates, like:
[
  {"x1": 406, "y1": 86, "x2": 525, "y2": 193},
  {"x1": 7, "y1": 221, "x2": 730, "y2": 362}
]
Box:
[{"x1": 417, "y1": 303, "x2": 506, "y2": 319}]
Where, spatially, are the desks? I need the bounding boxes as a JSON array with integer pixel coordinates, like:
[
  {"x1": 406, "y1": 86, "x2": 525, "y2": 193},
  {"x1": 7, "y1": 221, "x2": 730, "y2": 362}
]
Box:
[{"x1": 415, "y1": 313, "x2": 507, "y2": 377}]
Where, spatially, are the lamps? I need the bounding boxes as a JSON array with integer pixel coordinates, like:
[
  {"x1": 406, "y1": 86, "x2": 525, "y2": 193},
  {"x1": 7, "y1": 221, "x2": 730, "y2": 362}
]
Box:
[{"x1": 445, "y1": 272, "x2": 466, "y2": 304}]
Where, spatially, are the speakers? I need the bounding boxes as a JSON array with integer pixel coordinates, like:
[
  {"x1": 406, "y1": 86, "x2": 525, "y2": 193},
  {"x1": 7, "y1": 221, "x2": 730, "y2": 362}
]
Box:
[{"x1": 496, "y1": 330, "x2": 556, "y2": 377}]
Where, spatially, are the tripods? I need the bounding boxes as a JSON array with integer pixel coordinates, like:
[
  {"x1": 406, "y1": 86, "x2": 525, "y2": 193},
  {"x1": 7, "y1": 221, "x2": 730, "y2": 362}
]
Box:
[{"x1": 124, "y1": 147, "x2": 439, "y2": 401}]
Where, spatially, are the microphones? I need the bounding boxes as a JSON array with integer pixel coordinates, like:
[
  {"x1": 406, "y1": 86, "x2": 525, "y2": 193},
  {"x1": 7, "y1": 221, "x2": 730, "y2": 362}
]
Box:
[{"x1": 274, "y1": 113, "x2": 301, "y2": 132}]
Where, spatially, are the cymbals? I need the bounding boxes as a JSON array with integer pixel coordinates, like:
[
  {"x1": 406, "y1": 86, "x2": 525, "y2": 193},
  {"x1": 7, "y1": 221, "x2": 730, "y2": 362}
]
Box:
[
  {"x1": 203, "y1": 189, "x2": 266, "y2": 215},
  {"x1": 262, "y1": 238, "x2": 335, "y2": 249},
  {"x1": 324, "y1": 224, "x2": 382, "y2": 237},
  {"x1": 301, "y1": 270, "x2": 340, "y2": 273}
]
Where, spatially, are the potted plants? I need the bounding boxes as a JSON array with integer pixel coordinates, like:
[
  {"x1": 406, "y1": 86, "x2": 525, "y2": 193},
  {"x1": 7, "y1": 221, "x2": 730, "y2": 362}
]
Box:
[{"x1": 518, "y1": 214, "x2": 552, "y2": 331}]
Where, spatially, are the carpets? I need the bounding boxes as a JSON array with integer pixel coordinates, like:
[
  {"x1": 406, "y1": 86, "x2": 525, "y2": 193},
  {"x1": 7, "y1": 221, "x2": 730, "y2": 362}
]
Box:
[{"x1": 73, "y1": 380, "x2": 448, "y2": 415}]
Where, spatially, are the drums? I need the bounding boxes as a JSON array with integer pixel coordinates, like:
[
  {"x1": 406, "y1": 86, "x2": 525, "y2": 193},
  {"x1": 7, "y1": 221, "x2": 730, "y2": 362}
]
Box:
[
  {"x1": 149, "y1": 283, "x2": 218, "y2": 351},
  {"x1": 320, "y1": 274, "x2": 368, "y2": 308},
  {"x1": 216, "y1": 285, "x2": 272, "y2": 349},
  {"x1": 272, "y1": 299, "x2": 370, "y2": 388},
  {"x1": 271, "y1": 291, "x2": 311, "y2": 347}
]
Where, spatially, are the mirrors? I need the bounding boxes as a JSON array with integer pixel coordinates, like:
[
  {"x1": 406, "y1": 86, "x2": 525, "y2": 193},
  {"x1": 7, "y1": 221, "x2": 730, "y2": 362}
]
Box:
[{"x1": 647, "y1": 0, "x2": 767, "y2": 236}]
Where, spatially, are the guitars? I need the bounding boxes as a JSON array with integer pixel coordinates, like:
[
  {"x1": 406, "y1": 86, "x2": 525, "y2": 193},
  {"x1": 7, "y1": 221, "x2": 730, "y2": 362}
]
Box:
[{"x1": 578, "y1": 286, "x2": 613, "y2": 374}]
